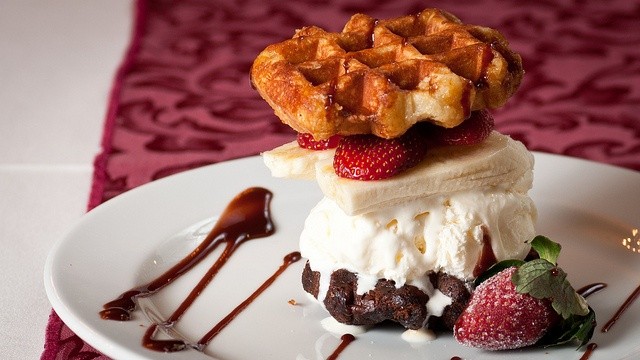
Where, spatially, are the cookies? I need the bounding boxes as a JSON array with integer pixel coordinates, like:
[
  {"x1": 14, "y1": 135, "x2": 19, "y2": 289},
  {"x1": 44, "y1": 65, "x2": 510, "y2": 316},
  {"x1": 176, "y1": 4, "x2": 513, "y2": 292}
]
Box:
[{"x1": 247, "y1": 6, "x2": 524, "y2": 139}]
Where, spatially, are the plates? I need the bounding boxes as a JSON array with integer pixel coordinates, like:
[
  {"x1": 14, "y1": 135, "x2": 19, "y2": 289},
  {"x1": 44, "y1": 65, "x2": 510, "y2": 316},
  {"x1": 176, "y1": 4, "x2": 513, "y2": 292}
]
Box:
[{"x1": 42, "y1": 149, "x2": 634, "y2": 358}]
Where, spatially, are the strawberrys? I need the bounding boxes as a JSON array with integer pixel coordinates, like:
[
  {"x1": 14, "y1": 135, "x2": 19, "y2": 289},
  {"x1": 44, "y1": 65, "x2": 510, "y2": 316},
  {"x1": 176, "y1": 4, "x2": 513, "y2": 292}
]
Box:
[
  {"x1": 452, "y1": 258, "x2": 579, "y2": 352},
  {"x1": 333, "y1": 133, "x2": 421, "y2": 181}
]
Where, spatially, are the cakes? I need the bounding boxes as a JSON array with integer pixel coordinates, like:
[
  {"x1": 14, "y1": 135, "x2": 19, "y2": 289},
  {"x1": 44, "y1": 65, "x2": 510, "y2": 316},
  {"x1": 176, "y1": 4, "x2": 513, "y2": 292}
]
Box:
[{"x1": 260, "y1": 129, "x2": 537, "y2": 332}]
